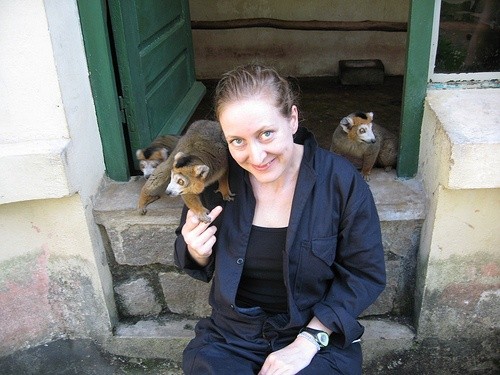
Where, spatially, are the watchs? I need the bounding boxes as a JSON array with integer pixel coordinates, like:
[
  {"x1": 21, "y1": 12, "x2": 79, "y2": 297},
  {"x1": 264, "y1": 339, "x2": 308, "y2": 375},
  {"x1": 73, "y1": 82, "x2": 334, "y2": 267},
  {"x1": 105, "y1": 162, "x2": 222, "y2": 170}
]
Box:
[{"x1": 300, "y1": 325, "x2": 329, "y2": 348}]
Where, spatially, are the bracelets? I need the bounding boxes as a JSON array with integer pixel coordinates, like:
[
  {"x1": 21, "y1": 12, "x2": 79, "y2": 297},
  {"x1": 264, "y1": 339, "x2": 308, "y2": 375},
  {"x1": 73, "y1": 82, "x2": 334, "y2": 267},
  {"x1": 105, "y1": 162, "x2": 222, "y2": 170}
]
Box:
[{"x1": 297, "y1": 332, "x2": 321, "y2": 350}]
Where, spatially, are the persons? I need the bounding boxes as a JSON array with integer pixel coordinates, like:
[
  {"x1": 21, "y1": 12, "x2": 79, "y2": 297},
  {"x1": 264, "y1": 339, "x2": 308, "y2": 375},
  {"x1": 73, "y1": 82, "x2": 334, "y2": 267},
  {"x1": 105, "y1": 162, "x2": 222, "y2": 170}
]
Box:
[{"x1": 172, "y1": 62, "x2": 387, "y2": 375}]
